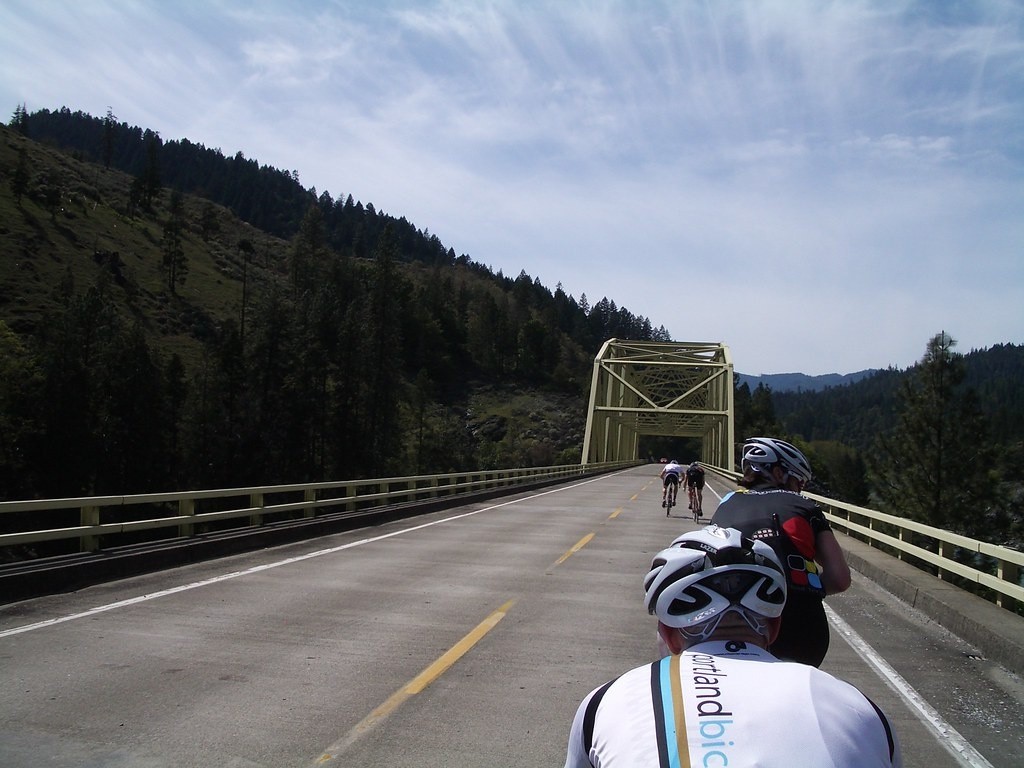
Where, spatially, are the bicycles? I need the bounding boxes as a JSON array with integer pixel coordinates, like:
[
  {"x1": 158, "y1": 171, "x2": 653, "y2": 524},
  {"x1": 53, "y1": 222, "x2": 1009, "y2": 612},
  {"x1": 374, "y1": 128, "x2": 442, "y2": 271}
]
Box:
[
  {"x1": 663, "y1": 478, "x2": 681, "y2": 517},
  {"x1": 683, "y1": 479, "x2": 702, "y2": 524}
]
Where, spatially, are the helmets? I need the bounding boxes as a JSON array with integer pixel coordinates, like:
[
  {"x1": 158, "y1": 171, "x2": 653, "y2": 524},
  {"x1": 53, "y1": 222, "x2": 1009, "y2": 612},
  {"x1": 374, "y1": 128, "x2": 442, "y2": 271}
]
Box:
[
  {"x1": 689, "y1": 461, "x2": 698, "y2": 467},
  {"x1": 740, "y1": 437, "x2": 812, "y2": 483},
  {"x1": 644, "y1": 525, "x2": 787, "y2": 628},
  {"x1": 670, "y1": 460, "x2": 678, "y2": 464}
]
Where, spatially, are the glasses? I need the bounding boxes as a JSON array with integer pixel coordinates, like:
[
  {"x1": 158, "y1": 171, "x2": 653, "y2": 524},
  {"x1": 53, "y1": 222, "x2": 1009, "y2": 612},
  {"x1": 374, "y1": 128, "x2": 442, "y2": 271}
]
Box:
[{"x1": 786, "y1": 470, "x2": 806, "y2": 493}]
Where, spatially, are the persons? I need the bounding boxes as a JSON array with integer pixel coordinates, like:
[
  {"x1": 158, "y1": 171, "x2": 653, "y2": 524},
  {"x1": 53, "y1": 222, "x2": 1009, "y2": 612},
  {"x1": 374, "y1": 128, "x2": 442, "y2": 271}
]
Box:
[
  {"x1": 707, "y1": 437, "x2": 852, "y2": 668},
  {"x1": 659, "y1": 460, "x2": 685, "y2": 508},
  {"x1": 564, "y1": 525, "x2": 903, "y2": 768},
  {"x1": 683, "y1": 462, "x2": 706, "y2": 517}
]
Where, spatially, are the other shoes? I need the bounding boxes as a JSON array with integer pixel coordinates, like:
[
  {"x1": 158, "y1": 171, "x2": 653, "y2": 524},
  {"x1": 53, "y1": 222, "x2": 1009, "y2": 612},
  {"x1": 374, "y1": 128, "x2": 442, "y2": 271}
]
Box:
[
  {"x1": 688, "y1": 502, "x2": 691, "y2": 509},
  {"x1": 698, "y1": 509, "x2": 702, "y2": 516},
  {"x1": 662, "y1": 500, "x2": 666, "y2": 508},
  {"x1": 672, "y1": 501, "x2": 676, "y2": 506}
]
